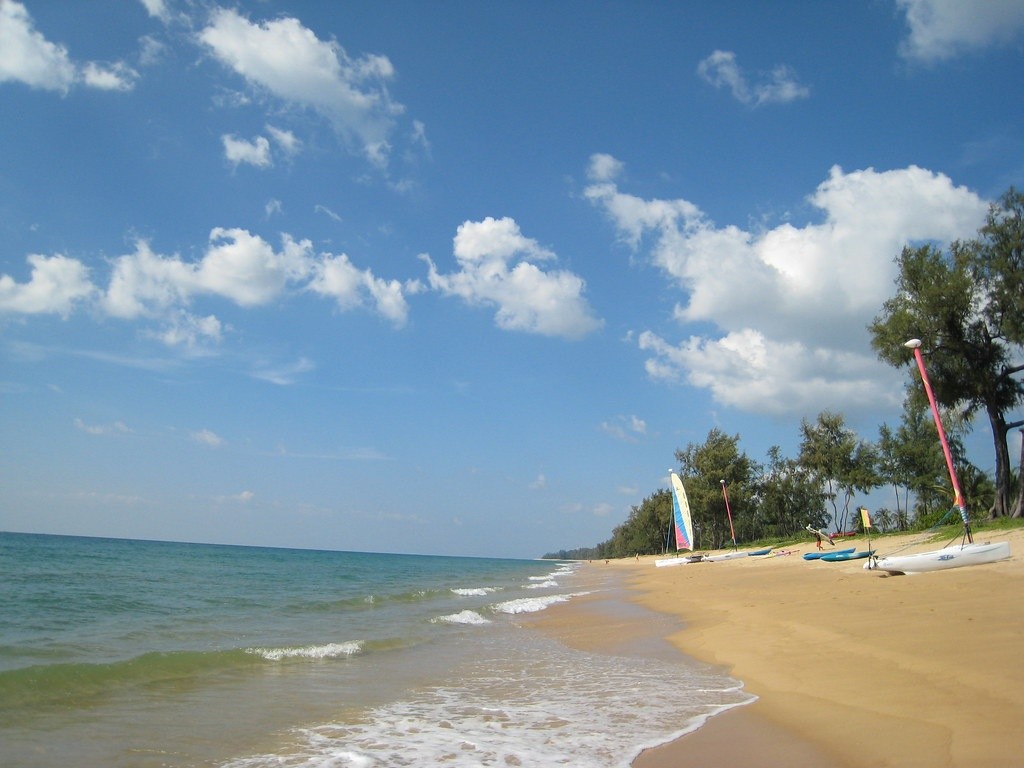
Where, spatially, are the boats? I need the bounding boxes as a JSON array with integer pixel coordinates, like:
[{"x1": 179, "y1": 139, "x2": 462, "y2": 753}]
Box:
[
  {"x1": 747, "y1": 548, "x2": 771, "y2": 556},
  {"x1": 862, "y1": 337, "x2": 1009, "y2": 574},
  {"x1": 821, "y1": 549, "x2": 877, "y2": 563},
  {"x1": 802, "y1": 547, "x2": 856, "y2": 561}
]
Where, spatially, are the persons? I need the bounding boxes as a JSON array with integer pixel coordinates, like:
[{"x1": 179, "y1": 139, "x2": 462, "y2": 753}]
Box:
[
  {"x1": 813, "y1": 530, "x2": 823, "y2": 551},
  {"x1": 634, "y1": 551, "x2": 640, "y2": 563}
]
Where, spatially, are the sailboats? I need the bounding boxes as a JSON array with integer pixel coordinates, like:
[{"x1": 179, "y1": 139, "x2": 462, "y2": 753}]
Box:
[{"x1": 655, "y1": 468, "x2": 748, "y2": 568}]
